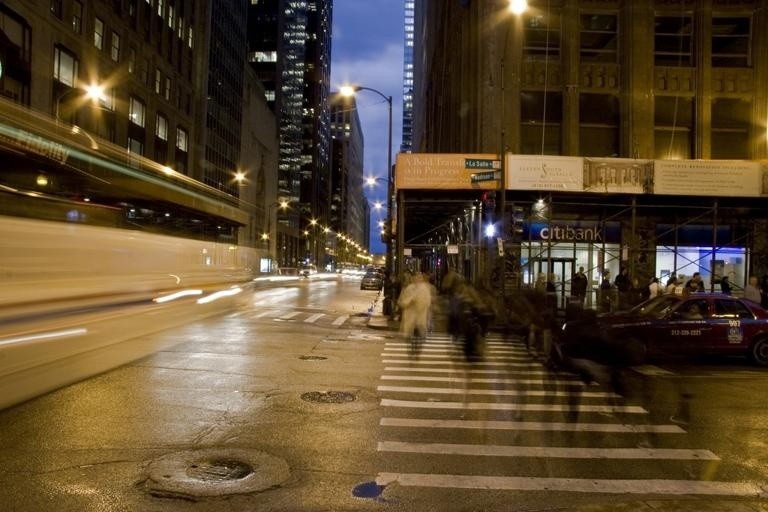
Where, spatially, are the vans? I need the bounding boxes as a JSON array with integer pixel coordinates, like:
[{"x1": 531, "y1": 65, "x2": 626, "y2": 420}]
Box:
[{"x1": 302, "y1": 264, "x2": 314, "y2": 276}]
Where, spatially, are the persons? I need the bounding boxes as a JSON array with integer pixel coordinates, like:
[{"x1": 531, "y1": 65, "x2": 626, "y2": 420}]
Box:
[{"x1": 377, "y1": 263, "x2": 768, "y2": 419}]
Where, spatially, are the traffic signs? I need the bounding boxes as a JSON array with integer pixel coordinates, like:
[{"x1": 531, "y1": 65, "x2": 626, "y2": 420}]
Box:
[
  {"x1": 465, "y1": 159, "x2": 501, "y2": 169},
  {"x1": 470, "y1": 171, "x2": 501, "y2": 182}
]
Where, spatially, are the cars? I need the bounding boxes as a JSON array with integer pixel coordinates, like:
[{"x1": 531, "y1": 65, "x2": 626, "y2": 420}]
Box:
[
  {"x1": 313, "y1": 265, "x2": 317, "y2": 274},
  {"x1": 554, "y1": 291, "x2": 767, "y2": 367},
  {"x1": 360, "y1": 265, "x2": 385, "y2": 290}
]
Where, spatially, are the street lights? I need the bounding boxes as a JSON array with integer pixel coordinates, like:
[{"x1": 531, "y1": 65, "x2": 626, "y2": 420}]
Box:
[
  {"x1": 317, "y1": 228, "x2": 329, "y2": 268},
  {"x1": 374, "y1": 202, "x2": 387, "y2": 210},
  {"x1": 499, "y1": 0, "x2": 529, "y2": 306},
  {"x1": 305, "y1": 220, "x2": 316, "y2": 264},
  {"x1": 367, "y1": 176, "x2": 389, "y2": 185},
  {"x1": 268, "y1": 202, "x2": 288, "y2": 258},
  {"x1": 338, "y1": 84, "x2": 393, "y2": 314}
]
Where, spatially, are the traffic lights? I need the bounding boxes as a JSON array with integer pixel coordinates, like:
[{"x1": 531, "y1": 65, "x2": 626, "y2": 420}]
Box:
[
  {"x1": 504, "y1": 202, "x2": 524, "y2": 243},
  {"x1": 437, "y1": 258, "x2": 444, "y2": 269}
]
